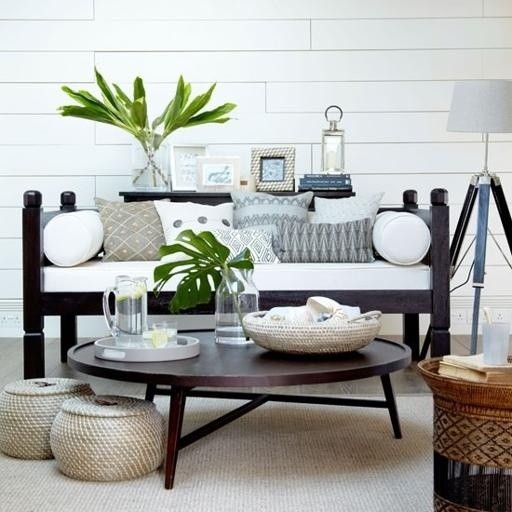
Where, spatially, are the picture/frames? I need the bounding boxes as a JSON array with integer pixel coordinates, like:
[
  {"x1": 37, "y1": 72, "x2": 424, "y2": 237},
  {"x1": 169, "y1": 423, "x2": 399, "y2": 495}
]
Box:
[
  {"x1": 170, "y1": 142, "x2": 210, "y2": 190},
  {"x1": 197, "y1": 157, "x2": 240, "y2": 190},
  {"x1": 251, "y1": 146, "x2": 294, "y2": 192}
]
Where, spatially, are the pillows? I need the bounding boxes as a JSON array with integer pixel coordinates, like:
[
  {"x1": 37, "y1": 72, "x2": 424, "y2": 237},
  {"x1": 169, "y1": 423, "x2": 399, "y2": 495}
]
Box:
[
  {"x1": 230, "y1": 191, "x2": 316, "y2": 226},
  {"x1": 154, "y1": 200, "x2": 234, "y2": 245},
  {"x1": 315, "y1": 192, "x2": 383, "y2": 222},
  {"x1": 43, "y1": 206, "x2": 101, "y2": 264},
  {"x1": 284, "y1": 218, "x2": 377, "y2": 265},
  {"x1": 95, "y1": 195, "x2": 168, "y2": 263},
  {"x1": 209, "y1": 226, "x2": 282, "y2": 266},
  {"x1": 373, "y1": 209, "x2": 433, "y2": 265}
]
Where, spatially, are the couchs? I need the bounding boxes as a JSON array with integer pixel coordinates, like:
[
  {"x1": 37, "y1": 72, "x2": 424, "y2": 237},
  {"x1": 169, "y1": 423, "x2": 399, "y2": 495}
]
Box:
[{"x1": 18, "y1": 190, "x2": 451, "y2": 376}]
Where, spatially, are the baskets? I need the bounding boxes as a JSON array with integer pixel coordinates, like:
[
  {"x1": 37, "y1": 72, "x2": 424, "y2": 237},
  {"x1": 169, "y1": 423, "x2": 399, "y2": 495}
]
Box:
[
  {"x1": 240, "y1": 305, "x2": 382, "y2": 354},
  {"x1": 417, "y1": 350, "x2": 512, "y2": 413}
]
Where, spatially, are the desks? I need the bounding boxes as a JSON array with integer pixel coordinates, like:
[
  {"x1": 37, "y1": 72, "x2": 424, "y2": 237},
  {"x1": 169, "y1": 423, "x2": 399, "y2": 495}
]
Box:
[
  {"x1": 416, "y1": 356, "x2": 512, "y2": 512},
  {"x1": 67, "y1": 327, "x2": 411, "y2": 489}
]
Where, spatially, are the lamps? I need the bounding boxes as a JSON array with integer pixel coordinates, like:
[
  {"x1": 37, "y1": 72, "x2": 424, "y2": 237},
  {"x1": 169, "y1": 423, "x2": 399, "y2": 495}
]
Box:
[{"x1": 414, "y1": 78, "x2": 512, "y2": 362}]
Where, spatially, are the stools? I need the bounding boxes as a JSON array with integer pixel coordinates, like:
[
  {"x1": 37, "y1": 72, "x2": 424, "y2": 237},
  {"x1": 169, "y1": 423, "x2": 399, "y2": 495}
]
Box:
[
  {"x1": 49, "y1": 395, "x2": 167, "y2": 484},
  {"x1": 1, "y1": 377, "x2": 95, "y2": 471}
]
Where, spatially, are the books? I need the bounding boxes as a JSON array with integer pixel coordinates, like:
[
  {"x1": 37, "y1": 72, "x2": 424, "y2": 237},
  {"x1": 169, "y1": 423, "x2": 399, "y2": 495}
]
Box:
[
  {"x1": 298, "y1": 173, "x2": 351, "y2": 190},
  {"x1": 439, "y1": 356, "x2": 511, "y2": 384}
]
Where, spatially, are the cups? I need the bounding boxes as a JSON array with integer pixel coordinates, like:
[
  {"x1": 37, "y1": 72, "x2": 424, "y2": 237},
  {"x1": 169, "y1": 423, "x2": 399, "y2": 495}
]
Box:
[
  {"x1": 483, "y1": 323, "x2": 511, "y2": 365},
  {"x1": 240, "y1": 174, "x2": 258, "y2": 193},
  {"x1": 146, "y1": 315, "x2": 178, "y2": 351},
  {"x1": 102, "y1": 274, "x2": 149, "y2": 349}
]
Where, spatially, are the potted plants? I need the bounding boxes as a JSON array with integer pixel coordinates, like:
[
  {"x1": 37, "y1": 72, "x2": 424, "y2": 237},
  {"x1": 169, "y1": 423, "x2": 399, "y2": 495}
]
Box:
[
  {"x1": 151, "y1": 229, "x2": 261, "y2": 349},
  {"x1": 62, "y1": 68, "x2": 232, "y2": 186}
]
